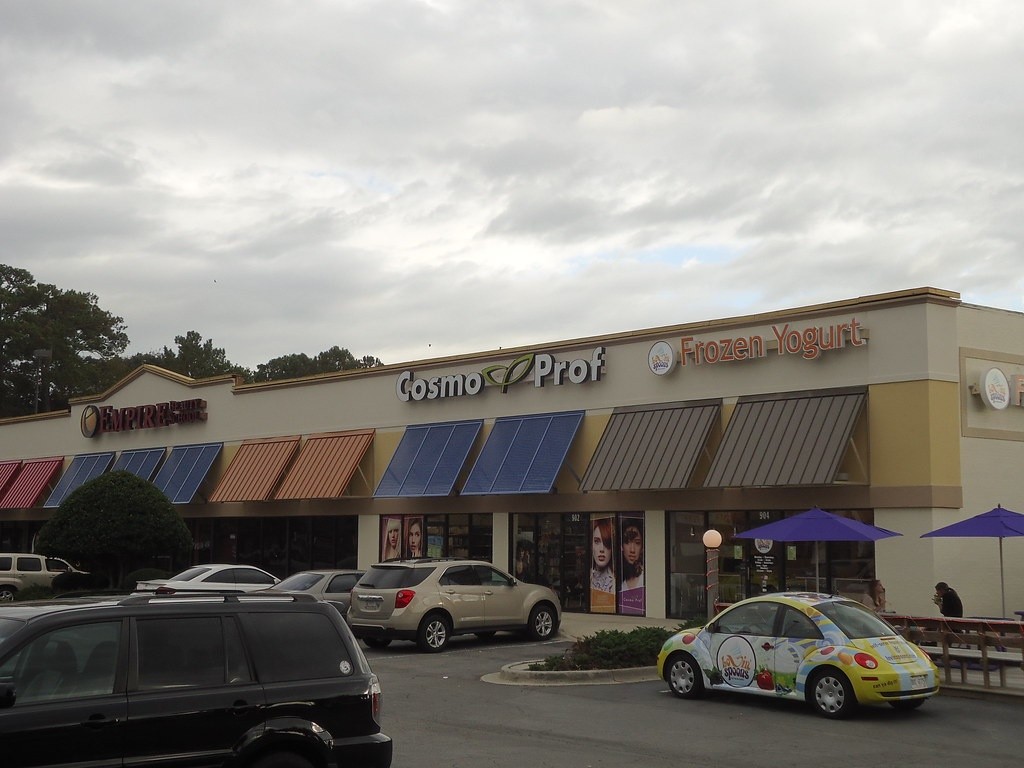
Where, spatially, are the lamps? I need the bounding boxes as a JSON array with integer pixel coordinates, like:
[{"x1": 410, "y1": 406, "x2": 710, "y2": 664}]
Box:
[
  {"x1": 702, "y1": 529, "x2": 722, "y2": 548},
  {"x1": 689, "y1": 526, "x2": 696, "y2": 537}
]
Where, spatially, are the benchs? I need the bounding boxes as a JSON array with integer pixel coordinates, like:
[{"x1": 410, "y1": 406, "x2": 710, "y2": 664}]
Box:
[{"x1": 881, "y1": 614, "x2": 1024, "y2": 696}]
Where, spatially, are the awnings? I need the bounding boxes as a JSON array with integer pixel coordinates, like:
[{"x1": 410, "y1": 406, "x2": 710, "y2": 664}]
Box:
[
  {"x1": 42, "y1": 451, "x2": 116, "y2": 508},
  {"x1": 460, "y1": 410, "x2": 587, "y2": 496},
  {"x1": 152, "y1": 442, "x2": 224, "y2": 505},
  {"x1": 701, "y1": 385, "x2": 871, "y2": 488},
  {"x1": 274, "y1": 428, "x2": 376, "y2": 500},
  {"x1": 370, "y1": 418, "x2": 484, "y2": 497},
  {"x1": 0, "y1": 456, "x2": 64, "y2": 509},
  {"x1": 208, "y1": 435, "x2": 302, "y2": 502},
  {"x1": 576, "y1": 396, "x2": 724, "y2": 491},
  {"x1": 109, "y1": 447, "x2": 167, "y2": 481}
]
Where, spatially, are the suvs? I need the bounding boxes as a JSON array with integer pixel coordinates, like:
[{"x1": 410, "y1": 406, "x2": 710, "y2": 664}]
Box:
[
  {"x1": 0, "y1": 585, "x2": 393, "y2": 768},
  {"x1": 0, "y1": 553, "x2": 95, "y2": 602},
  {"x1": 342, "y1": 555, "x2": 561, "y2": 654}
]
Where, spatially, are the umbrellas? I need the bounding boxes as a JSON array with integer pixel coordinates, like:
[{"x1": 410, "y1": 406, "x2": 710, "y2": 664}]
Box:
[
  {"x1": 919, "y1": 502, "x2": 1024, "y2": 617},
  {"x1": 731, "y1": 505, "x2": 904, "y2": 592}
]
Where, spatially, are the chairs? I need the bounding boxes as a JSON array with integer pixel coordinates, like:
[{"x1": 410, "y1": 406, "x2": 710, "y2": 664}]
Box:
[
  {"x1": 70, "y1": 641, "x2": 119, "y2": 696},
  {"x1": 20, "y1": 640, "x2": 77, "y2": 701}
]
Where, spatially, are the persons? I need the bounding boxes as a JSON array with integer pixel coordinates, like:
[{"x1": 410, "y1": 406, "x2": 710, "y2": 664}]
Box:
[
  {"x1": 618, "y1": 519, "x2": 644, "y2": 614},
  {"x1": 922, "y1": 582, "x2": 963, "y2": 661},
  {"x1": 407, "y1": 518, "x2": 422, "y2": 557},
  {"x1": 862, "y1": 580, "x2": 886, "y2": 613},
  {"x1": 739, "y1": 608, "x2": 762, "y2": 632},
  {"x1": 592, "y1": 519, "x2": 615, "y2": 594},
  {"x1": 384, "y1": 518, "x2": 402, "y2": 560},
  {"x1": 515, "y1": 543, "x2": 530, "y2": 582}
]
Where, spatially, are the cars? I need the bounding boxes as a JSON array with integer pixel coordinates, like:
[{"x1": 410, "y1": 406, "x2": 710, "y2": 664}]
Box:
[
  {"x1": 245, "y1": 569, "x2": 365, "y2": 623},
  {"x1": 656, "y1": 591, "x2": 942, "y2": 717},
  {"x1": 133, "y1": 563, "x2": 283, "y2": 603}
]
